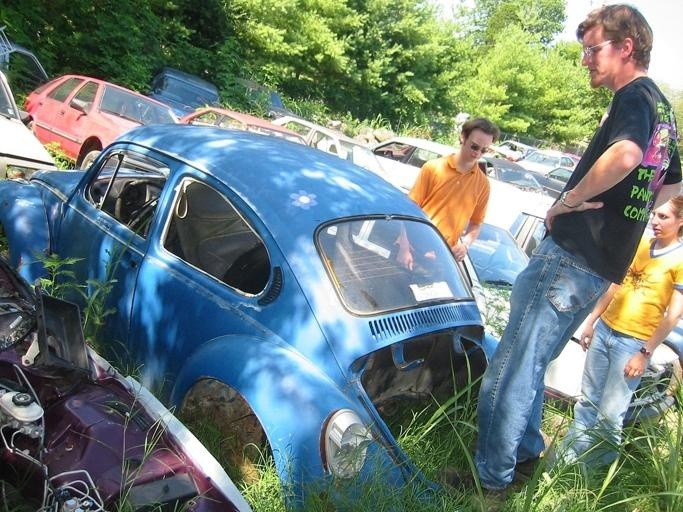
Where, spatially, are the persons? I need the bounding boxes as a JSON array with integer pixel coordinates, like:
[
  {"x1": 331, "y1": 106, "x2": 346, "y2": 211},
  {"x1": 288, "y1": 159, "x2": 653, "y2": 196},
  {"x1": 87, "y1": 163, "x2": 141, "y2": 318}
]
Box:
[
  {"x1": 445, "y1": 5, "x2": 678, "y2": 495},
  {"x1": 391, "y1": 118, "x2": 497, "y2": 276},
  {"x1": 559, "y1": 193, "x2": 682, "y2": 479}
]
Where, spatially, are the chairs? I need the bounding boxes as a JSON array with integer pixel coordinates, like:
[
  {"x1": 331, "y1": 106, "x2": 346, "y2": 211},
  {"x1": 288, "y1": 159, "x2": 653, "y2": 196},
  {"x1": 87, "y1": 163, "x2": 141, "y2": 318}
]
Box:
[{"x1": 173, "y1": 178, "x2": 268, "y2": 295}]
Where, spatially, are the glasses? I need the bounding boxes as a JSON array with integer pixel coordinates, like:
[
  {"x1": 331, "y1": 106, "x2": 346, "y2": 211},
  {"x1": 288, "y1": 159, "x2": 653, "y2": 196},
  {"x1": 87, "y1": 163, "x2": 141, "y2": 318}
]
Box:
[
  {"x1": 466, "y1": 140, "x2": 490, "y2": 154},
  {"x1": 581, "y1": 39, "x2": 614, "y2": 56}
]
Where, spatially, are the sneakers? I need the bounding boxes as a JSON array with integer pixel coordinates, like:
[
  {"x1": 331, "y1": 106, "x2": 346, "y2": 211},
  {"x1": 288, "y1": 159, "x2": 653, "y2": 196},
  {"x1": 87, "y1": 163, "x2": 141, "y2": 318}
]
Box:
[
  {"x1": 514, "y1": 458, "x2": 542, "y2": 480},
  {"x1": 441, "y1": 470, "x2": 508, "y2": 503}
]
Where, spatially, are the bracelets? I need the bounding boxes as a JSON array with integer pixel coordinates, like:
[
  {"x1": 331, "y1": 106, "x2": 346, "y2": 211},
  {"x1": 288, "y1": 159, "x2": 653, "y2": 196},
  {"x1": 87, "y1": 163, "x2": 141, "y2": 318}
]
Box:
[{"x1": 463, "y1": 243, "x2": 469, "y2": 250}]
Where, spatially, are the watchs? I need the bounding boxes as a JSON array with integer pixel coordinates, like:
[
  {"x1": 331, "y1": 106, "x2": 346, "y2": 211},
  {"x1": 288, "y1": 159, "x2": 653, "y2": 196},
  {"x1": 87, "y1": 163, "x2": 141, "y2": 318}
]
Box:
[
  {"x1": 559, "y1": 189, "x2": 583, "y2": 208},
  {"x1": 640, "y1": 346, "x2": 652, "y2": 358}
]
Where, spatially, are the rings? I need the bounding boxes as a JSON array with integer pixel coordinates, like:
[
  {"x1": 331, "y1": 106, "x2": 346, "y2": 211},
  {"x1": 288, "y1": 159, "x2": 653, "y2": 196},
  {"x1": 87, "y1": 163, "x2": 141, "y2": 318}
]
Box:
[
  {"x1": 634, "y1": 372, "x2": 639, "y2": 376},
  {"x1": 459, "y1": 256, "x2": 463, "y2": 260}
]
